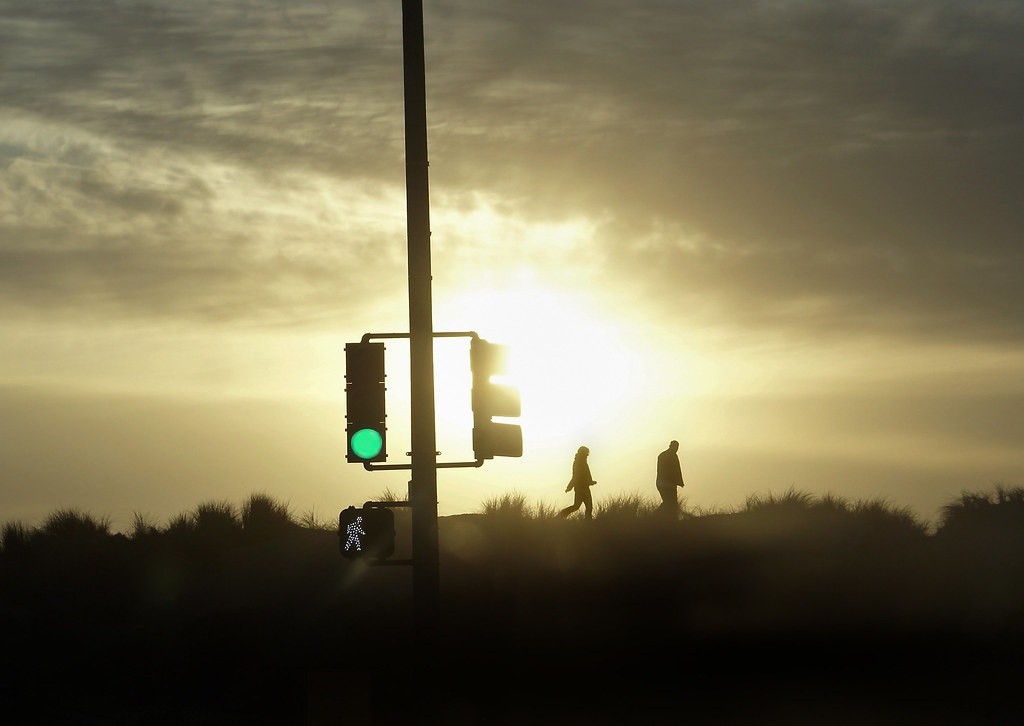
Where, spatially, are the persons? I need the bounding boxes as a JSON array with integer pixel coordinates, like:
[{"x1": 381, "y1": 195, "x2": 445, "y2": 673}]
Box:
[
  {"x1": 655, "y1": 441, "x2": 685, "y2": 512},
  {"x1": 558, "y1": 446, "x2": 596, "y2": 519}
]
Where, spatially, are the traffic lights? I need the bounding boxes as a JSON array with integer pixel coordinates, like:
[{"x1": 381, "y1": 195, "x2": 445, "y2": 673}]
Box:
[
  {"x1": 338, "y1": 506, "x2": 394, "y2": 561},
  {"x1": 470, "y1": 337, "x2": 524, "y2": 458},
  {"x1": 343, "y1": 341, "x2": 389, "y2": 463}
]
